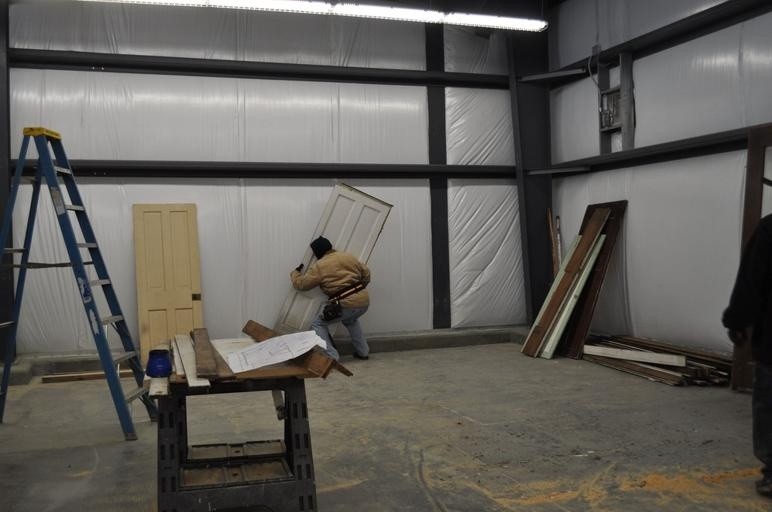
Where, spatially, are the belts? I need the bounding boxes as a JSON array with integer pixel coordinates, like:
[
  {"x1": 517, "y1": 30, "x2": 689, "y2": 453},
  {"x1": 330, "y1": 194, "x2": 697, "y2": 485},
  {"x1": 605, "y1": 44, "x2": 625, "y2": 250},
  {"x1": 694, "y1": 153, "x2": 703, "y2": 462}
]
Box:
[{"x1": 328, "y1": 283, "x2": 364, "y2": 303}]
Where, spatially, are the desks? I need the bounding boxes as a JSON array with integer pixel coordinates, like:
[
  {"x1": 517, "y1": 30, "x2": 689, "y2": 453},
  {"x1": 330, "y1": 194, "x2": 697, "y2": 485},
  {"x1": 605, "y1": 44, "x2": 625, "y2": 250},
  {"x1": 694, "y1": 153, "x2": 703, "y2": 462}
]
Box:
[{"x1": 149, "y1": 336, "x2": 317, "y2": 511}]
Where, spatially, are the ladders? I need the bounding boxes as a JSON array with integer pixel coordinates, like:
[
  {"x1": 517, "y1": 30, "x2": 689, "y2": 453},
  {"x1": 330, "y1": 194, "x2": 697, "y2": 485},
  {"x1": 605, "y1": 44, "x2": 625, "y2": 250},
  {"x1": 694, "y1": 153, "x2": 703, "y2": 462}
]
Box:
[{"x1": 2, "y1": 126, "x2": 161, "y2": 441}]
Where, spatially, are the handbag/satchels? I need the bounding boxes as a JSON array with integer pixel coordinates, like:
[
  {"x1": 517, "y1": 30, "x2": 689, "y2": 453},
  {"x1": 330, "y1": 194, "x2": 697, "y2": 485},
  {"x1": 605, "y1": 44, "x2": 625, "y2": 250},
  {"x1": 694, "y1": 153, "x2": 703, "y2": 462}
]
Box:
[{"x1": 323, "y1": 302, "x2": 343, "y2": 321}]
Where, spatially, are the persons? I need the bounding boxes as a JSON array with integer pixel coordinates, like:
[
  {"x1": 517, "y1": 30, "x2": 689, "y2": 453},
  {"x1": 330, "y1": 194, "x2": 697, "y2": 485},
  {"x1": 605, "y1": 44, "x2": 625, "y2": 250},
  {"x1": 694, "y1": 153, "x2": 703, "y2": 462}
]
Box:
[
  {"x1": 289, "y1": 238, "x2": 374, "y2": 363},
  {"x1": 720, "y1": 212, "x2": 772, "y2": 495}
]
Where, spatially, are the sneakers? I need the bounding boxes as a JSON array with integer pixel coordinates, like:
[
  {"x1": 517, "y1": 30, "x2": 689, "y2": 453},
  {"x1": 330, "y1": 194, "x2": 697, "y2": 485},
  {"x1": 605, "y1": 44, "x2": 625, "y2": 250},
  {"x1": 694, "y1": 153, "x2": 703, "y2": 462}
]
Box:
[{"x1": 352, "y1": 351, "x2": 368, "y2": 360}]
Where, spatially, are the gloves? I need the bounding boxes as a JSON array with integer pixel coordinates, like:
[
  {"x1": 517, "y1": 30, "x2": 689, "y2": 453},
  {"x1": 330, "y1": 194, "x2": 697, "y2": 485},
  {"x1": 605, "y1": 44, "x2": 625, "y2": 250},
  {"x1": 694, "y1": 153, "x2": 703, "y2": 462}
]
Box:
[{"x1": 296, "y1": 263, "x2": 303, "y2": 272}]
